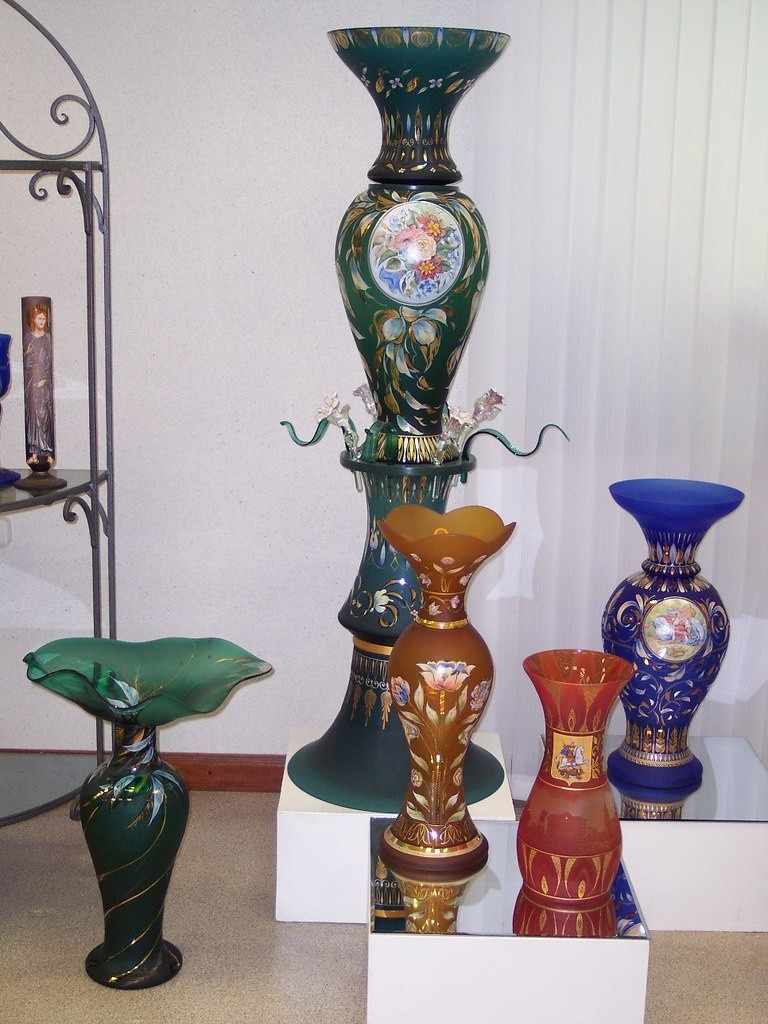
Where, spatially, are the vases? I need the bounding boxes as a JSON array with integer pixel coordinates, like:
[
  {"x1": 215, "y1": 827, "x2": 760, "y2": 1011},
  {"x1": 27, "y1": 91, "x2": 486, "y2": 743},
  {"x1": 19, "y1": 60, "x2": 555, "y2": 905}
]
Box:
[
  {"x1": 22, "y1": 637, "x2": 272, "y2": 990},
  {"x1": 515, "y1": 649, "x2": 634, "y2": 907},
  {"x1": 375, "y1": 503, "x2": 518, "y2": 872},
  {"x1": 277, "y1": 27, "x2": 571, "y2": 813},
  {"x1": 601, "y1": 477, "x2": 745, "y2": 789}
]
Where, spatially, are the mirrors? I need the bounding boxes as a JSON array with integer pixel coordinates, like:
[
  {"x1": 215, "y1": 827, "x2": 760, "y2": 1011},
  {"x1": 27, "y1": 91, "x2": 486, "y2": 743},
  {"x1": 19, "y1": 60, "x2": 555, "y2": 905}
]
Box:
[
  {"x1": 540, "y1": 734, "x2": 768, "y2": 823},
  {"x1": 371, "y1": 816, "x2": 652, "y2": 939}
]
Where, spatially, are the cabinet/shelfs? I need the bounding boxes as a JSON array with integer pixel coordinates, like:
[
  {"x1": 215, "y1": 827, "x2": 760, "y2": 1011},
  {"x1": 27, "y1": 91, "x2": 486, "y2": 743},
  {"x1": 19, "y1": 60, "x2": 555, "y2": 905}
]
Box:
[{"x1": 0, "y1": 0, "x2": 116, "y2": 827}]
